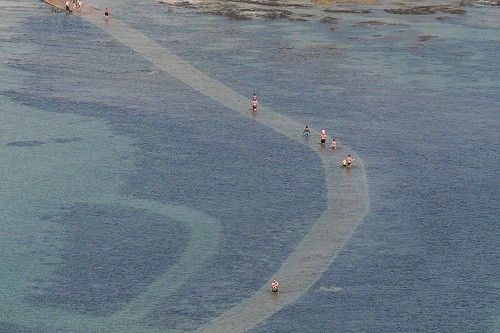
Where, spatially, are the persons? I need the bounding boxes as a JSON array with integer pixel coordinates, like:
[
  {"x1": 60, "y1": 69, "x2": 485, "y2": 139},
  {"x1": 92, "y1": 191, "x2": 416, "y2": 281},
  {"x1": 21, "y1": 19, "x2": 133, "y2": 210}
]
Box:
[
  {"x1": 319, "y1": 130, "x2": 327, "y2": 145},
  {"x1": 251, "y1": 94, "x2": 258, "y2": 111},
  {"x1": 65, "y1": 1, "x2": 70, "y2": 13},
  {"x1": 347, "y1": 155, "x2": 353, "y2": 168},
  {"x1": 303, "y1": 126, "x2": 311, "y2": 137},
  {"x1": 342, "y1": 158, "x2": 346, "y2": 167},
  {"x1": 271, "y1": 280, "x2": 279, "y2": 293},
  {"x1": 104, "y1": 7, "x2": 109, "y2": 18},
  {"x1": 331, "y1": 139, "x2": 337, "y2": 149}
]
